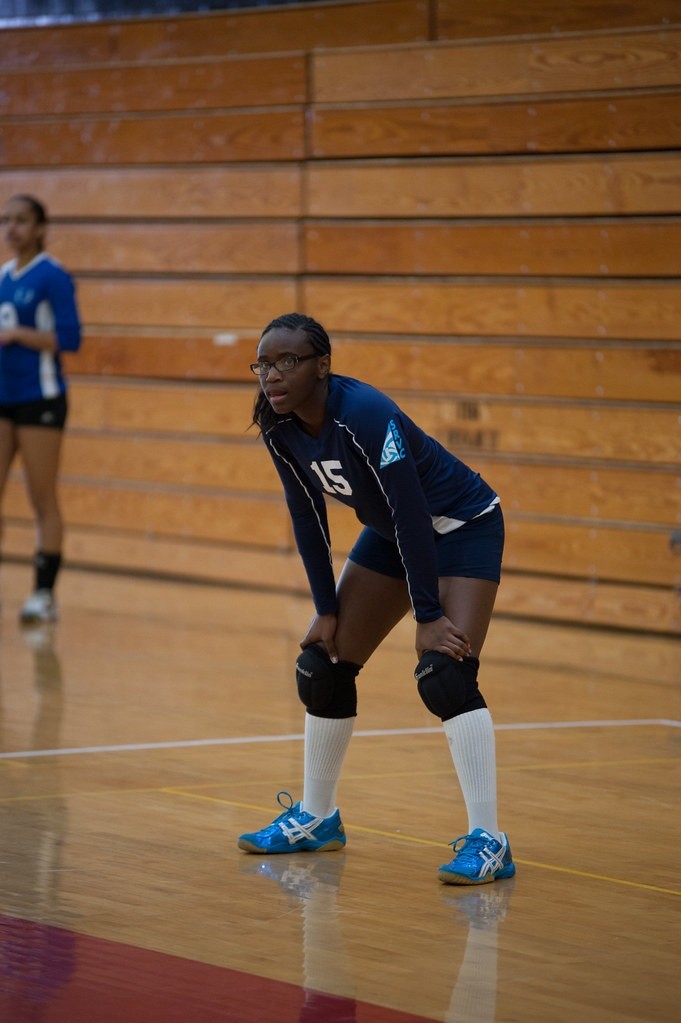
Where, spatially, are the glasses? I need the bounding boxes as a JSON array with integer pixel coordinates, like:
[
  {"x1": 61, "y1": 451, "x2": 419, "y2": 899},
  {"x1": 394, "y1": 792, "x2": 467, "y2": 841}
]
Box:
[{"x1": 249, "y1": 354, "x2": 317, "y2": 375}]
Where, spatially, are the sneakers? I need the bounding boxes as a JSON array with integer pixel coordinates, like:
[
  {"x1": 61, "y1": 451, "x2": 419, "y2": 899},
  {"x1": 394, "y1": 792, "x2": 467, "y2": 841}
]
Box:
[
  {"x1": 438, "y1": 828, "x2": 516, "y2": 884},
  {"x1": 21, "y1": 589, "x2": 58, "y2": 621},
  {"x1": 238, "y1": 792, "x2": 346, "y2": 854},
  {"x1": 240, "y1": 855, "x2": 344, "y2": 901},
  {"x1": 442, "y1": 887, "x2": 511, "y2": 924}
]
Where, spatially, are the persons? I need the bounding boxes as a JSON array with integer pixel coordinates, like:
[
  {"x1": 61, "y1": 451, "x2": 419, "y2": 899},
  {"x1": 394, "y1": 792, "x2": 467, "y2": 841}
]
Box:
[
  {"x1": 0, "y1": 193, "x2": 81, "y2": 625},
  {"x1": 237, "y1": 312, "x2": 517, "y2": 885}
]
